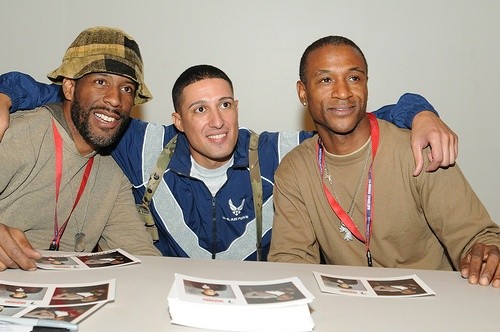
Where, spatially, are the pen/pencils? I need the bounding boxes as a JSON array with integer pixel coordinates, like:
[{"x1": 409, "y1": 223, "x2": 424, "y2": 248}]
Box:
[
  {"x1": 49, "y1": 241, "x2": 59, "y2": 250},
  {"x1": 367, "y1": 250, "x2": 373, "y2": 267}
]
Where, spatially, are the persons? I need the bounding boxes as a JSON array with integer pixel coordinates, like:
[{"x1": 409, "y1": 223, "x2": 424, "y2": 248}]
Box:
[
  {"x1": 75, "y1": 255, "x2": 128, "y2": 267},
  {"x1": 22, "y1": 307, "x2": 84, "y2": 321},
  {"x1": 41, "y1": 257, "x2": 69, "y2": 265},
  {"x1": 5, "y1": 285, "x2": 43, "y2": 299},
  {"x1": 52, "y1": 287, "x2": 108, "y2": 303},
  {"x1": 240, "y1": 285, "x2": 296, "y2": 302},
  {"x1": 0, "y1": 26, "x2": 162, "y2": 272},
  {"x1": 322, "y1": 276, "x2": 359, "y2": 291},
  {"x1": 368, "y1": 279, "x2": 418, "y2": 295},
  {"x1": 184, "y1": 280, "x2": 227, "y2": 296},
  {"x1": 267, "y1": 35, "x2": 500, "y2": 289},
  {"x1": 0, "y1": 65, "x2": 458, "y2": 262}
]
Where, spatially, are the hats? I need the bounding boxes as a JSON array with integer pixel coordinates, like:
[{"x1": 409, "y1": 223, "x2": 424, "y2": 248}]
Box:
[{"x1": 47, "y1": 25, "x2": 153, "y2": 106}]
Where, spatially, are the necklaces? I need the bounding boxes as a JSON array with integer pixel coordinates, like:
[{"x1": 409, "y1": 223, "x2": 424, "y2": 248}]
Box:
[
  {"x1": 319, "y1": 140, "x2": 372, "y2": 242},
  {"x1": 63, "y1": 140, "x2": 101, "y2": 251}
]
